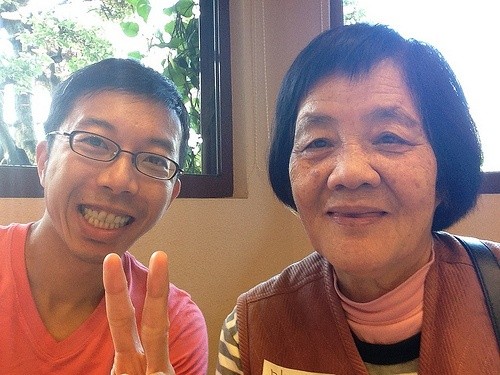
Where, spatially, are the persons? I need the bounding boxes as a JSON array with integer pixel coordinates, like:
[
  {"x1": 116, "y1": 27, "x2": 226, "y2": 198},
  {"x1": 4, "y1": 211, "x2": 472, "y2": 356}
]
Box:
[
  {"x1": 0, "y1": 59, "x2": 209, "y2": 375},
  {"x1": 215, "y1": 22, "x2": 500, "y2": 375}
]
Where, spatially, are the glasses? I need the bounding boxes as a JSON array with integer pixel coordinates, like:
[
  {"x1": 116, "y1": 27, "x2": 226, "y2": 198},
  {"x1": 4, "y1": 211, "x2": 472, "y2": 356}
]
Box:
[{"x1": 45, "y1": 130, "x2": 186, "y2": 180}]
